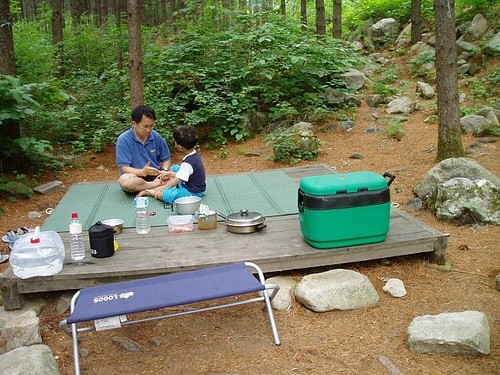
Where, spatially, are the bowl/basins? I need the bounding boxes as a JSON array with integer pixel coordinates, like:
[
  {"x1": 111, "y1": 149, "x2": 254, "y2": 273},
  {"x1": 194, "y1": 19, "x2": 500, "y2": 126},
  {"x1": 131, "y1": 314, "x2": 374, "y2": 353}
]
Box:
[
  {"x1": 167, "y1": 215, "x2": 194, "y2": 233},
  {"x1": 174, "y1": 196, "x2": 202, "y2": 214},
  {"x1": 101, "y1": 219, "x2": 125, "y2": 234}
]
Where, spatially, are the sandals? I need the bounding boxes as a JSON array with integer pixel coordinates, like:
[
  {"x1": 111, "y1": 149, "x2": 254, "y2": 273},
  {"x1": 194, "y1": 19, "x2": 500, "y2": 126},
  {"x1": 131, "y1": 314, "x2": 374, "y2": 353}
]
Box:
[{"x1": 1, "y1": 226, "x2": 36, "y2": 250}]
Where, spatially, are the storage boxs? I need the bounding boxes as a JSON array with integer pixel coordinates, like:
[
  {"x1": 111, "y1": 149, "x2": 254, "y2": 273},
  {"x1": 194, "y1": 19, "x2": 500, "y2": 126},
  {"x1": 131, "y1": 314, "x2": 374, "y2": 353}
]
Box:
[
  {"x1": 295, "y1": 169, "x2": 397, "y2": 251},
  {"x1": 167, "y1": 214, "x2": 193, "y2": 233}
]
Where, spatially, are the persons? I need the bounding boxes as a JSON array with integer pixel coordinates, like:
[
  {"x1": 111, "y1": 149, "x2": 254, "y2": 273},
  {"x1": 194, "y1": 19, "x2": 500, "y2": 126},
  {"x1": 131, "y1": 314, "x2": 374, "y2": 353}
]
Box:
[
  {"x1": 116, "y1": 106, "x2": 170, "y2": 193},
  {"x1": 135, "y1": 125, "x2": 206, "y2": 203}
]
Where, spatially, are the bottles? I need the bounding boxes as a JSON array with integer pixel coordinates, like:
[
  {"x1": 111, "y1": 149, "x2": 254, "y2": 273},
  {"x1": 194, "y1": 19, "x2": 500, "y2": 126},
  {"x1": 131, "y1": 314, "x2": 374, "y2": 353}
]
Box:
[
  {"x1": 134, "y1": 190, "x2": 150, "y2": 234},
  {"x1": 170, "y1": 164, "x2": 180, "y2": 172},
  {"x1": 69, "y1": 213, "x2": 85, "y2": 260}
]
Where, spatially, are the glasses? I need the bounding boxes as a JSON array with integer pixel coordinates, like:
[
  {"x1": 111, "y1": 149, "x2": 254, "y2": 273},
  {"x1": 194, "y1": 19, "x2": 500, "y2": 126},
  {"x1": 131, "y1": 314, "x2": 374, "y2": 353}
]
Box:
[{"x1": 139, "y1": 122, "x2": 156, "y2": 129}]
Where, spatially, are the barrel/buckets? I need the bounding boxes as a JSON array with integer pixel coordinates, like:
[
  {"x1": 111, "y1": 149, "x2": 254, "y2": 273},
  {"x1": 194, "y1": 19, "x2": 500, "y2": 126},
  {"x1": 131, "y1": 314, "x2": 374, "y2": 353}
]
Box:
[
  {"x1": 9, "y1": 226, "x2": 65, "y2": 279},
  {"x1": 89, "y1": 221, "x2": 114, "y2": 258}
]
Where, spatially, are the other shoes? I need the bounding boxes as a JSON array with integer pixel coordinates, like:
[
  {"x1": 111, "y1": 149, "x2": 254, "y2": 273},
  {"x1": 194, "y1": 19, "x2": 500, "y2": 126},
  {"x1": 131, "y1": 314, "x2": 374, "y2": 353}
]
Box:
[{"x1": 0, "y1": 253, "x2": 9, "y2": 263}]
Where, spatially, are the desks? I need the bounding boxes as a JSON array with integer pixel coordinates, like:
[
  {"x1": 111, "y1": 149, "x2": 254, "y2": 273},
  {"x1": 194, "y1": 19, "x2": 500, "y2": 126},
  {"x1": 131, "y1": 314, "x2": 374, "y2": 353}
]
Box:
[{"x1": 59, "y1": 262, "x2": 279, "y2": 375}]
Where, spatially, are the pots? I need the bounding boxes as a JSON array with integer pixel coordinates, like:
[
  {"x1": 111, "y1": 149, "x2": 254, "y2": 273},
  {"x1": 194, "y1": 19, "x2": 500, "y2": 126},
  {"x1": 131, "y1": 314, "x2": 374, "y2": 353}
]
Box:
[{"x1": 216, "y1": 209, "x2": 267, "y2": 234}]
